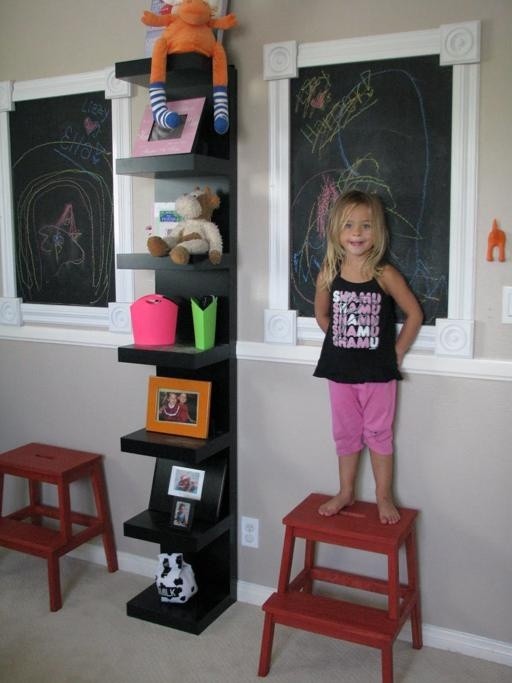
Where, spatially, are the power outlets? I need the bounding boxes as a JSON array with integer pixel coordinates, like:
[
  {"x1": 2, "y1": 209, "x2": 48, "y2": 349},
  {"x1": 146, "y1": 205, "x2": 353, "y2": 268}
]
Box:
[{"x1": 240, "y1": 516, "x2": 259, "y2": 549}]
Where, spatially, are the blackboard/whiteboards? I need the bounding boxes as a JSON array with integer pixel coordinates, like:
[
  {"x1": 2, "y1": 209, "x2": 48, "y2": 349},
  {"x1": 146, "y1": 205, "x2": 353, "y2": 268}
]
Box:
[
  {"x1": 262, "y1": 21, "x2": 481, "y2": 360},
  {"x1": 0, "y1": 66, "x2": 135, "y2": 334}
]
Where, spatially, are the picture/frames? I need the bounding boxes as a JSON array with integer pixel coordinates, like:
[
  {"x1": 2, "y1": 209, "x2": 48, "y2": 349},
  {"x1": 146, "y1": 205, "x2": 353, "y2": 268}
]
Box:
[
  {"x1": 168, "y1": 496, "x2": 197, "y2": 532},
  {"x1": 146, "y1": 375, "x2": 212, "y2": 439},
  {"x1": 129, "y1": 95, "x2": 206, "y2": 157},
  {"x1": 148, "y1": 458, "x2": 229, "y2": 521}
]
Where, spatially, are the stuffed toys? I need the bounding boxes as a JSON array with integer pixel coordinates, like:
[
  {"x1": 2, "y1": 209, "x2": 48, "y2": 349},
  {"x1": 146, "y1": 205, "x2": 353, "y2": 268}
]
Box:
[
  {"x1": 139, "y1": 0, "x2": 240, "y2": 135},
  {"x1": 145, "y1": 183, "x2": 224, "y2": 266}
]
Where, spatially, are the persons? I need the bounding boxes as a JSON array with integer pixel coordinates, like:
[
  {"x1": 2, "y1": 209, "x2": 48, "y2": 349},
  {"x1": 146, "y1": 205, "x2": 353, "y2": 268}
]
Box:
[
  {"x1": 159, "y1": 392, "x2": 187, "y2": 424},
  {"x1": 174, "y1": 502, "x2": 187, "y2": 524},
  {"x1": 309, "y1": 187, "x2": 426, "y2": 527},
  {"x1": 177, "y1": 392, "x2": 196, "y2": 423}
]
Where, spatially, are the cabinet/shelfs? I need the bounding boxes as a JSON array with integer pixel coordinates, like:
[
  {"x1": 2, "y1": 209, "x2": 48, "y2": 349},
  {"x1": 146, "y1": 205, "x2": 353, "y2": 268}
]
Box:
[{"x1": 115, "y1": 54, "x2": 238, "y2": 634}]
